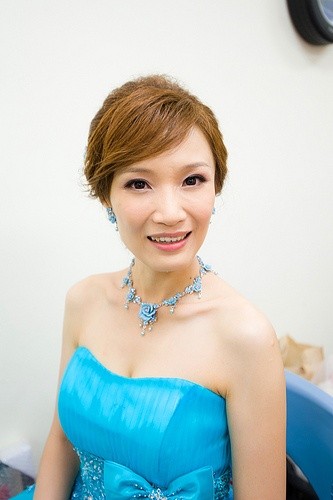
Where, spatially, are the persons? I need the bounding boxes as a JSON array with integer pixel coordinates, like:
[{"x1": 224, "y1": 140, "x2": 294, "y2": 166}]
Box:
[{"x1": 9, "y1": 75, "x2": 286, "y2": 500}]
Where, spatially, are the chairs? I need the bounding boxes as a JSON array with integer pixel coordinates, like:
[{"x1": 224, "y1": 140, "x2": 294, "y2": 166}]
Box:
[{"x1": 285, "y1": 369, "x2": 333, "y2": 500}]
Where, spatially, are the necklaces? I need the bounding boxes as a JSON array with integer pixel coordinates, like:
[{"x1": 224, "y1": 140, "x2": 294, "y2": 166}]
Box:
[{"x1": 121, "y1": 255, "x2": 219, "y2": 336}]
